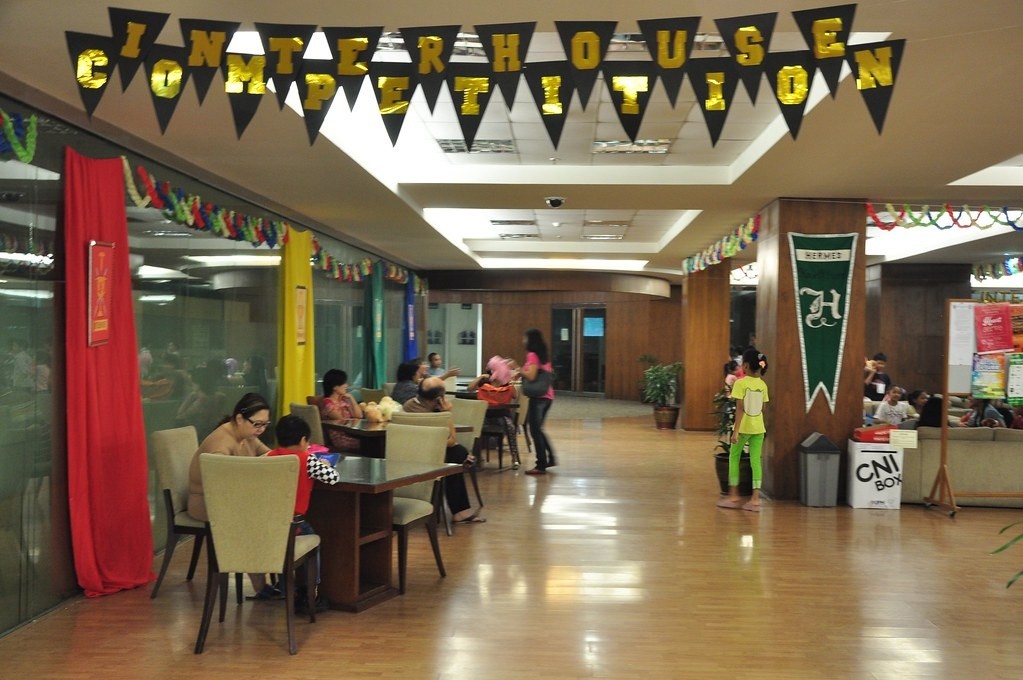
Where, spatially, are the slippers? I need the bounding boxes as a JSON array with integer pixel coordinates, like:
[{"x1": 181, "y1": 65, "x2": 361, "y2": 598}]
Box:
[
  {"x1": 453, "y1": 513, "x2": 487, "y2": 525},
  {"x1": 245, "y1": 583, "x2": 287, "y2": 600}
]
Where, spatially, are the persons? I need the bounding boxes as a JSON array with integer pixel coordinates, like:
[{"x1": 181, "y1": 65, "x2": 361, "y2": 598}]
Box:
[
  {"x1": 863, "y1": 354, "x2": 1023, "y2": 430},
  {"x1": 187, "y1": 352, "x2": 526, "y2": 611},
  {"x1": 517, "y1": 329, "x2": 559, "y2": 475},
  {"x1": 715, "y1": 350, "x2": 768, "y2": 511},
  {"x1": 724, "y1": 333, "x2": 757, "y2": 428}
]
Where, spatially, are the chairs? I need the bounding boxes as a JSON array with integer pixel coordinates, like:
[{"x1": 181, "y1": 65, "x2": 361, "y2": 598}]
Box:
[
  {"x1": 149, "y1": 425, "x2": 243, "y2": 605},
  {"x1": 217, "y1": 386, "x2": 261, "y2": 417},
  {"x1": 143, "y1": 400, "x2": 181, "y2": 457},
  {"x1": 361, "y1": 388, "x2": 389, "y2": 406},
  {"x1": 434, "y1": 374, "x2": 457, "y2": 401},
  {"x1": 289, "y1": 402, "x2": 327, "y2": 447},
  {"x1": 392, "y1": 411, "x2": 455, "y2": 427},
  {"x1": 382, "y1": 382, "x2": 396, "y2": 397},
  {"x1": 385, "y1": 421, "x2": 451, "y2": 596},
  {"x1": 306, "y1": 394, "x2": 326, "y2": 420},
  {"x1": 482, "y1": 424, "x2": 508, "y2": 469},
  {"x1": 451, "y1": 397, "x2": 489, "y2": 508},
  {"x1": 511, "y1": 380, "x2": 532, "y2": 453},
  {"x1": 193, "y1": 452, "x2": 322, "y2": 659}
]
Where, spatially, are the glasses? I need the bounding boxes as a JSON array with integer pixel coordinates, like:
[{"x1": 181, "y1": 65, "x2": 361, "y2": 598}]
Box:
[{"x1": 246, "y1": 419, "x2": 271, "y2": 428}]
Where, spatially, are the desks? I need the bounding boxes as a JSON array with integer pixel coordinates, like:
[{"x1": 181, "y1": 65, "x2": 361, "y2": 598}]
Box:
[
  {"x1": 446, "y1": 385, "x2": 479, "y2": 400},
  {"x1": 307, "y1": 454, "x2": 467, "y2": 616},
  {"x1": 322, "y1": 418, "x2": 475, "y2": 458}
]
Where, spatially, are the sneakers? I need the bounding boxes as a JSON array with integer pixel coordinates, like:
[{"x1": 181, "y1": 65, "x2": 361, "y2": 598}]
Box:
[
  {"x1": 742, "y1": 501, "x2": 760, "y2": 511},
  {"x1": 715, "y1": 498, "x2": 740, "y2": 508},
  {"x1": 295, "y1": 594, "x2": 330, "y2": 613}
]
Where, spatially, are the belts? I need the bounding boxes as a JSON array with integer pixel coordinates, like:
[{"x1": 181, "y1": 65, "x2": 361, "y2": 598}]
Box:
[{"x1": 293, "y1": 514, "x2": 305, "y2": 523}]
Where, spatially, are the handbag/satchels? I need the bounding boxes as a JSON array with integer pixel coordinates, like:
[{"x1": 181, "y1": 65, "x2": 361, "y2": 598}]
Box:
[{"x1": 522, "y1": 368, "x2": 557, "y2": 398}]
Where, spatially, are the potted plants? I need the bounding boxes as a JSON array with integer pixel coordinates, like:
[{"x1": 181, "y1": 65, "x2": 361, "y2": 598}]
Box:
[
  {"x1": 641, "y1": 361, "x2": 685, "y2": 430},
  {"x1": 635, "y1": 352, "x2": 656, "y2": 401},
  {"x1": 705, "y1": 381, "x2": 768, "y2": 497}
]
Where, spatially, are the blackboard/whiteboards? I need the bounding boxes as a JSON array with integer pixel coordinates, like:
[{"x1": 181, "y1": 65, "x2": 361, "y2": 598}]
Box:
[{"x1": 948, "y1": 298, "x2": 1002, "y2": 393}]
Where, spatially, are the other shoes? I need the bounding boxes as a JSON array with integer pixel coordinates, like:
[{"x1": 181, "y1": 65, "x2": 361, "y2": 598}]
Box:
[
  {"x1": 535, "y1": 462, "x2": 555, "y2": 469},
  {"x1": 524, "y1": 468, "x2": 547, "y2": 474}
]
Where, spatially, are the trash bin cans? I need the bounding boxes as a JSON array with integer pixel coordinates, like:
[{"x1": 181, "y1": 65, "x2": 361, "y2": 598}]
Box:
[{"x1": 800, "y1": 432, "x2": 842, "y2": 506}]
